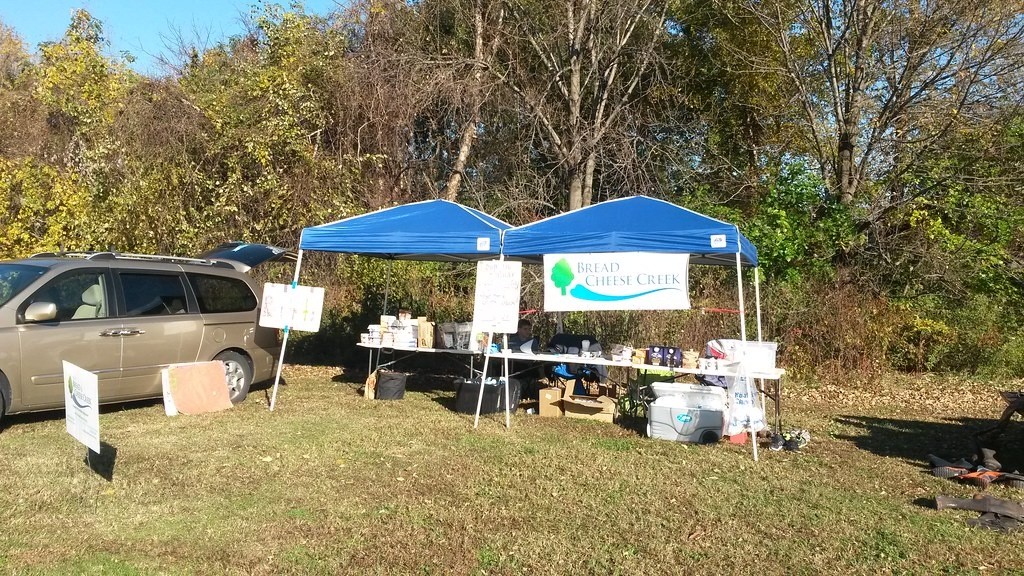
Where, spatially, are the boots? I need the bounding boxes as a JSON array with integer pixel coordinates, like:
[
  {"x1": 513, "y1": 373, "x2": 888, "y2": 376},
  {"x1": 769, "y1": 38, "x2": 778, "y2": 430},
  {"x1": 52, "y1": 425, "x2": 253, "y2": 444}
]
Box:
[{"x1": 929, "y1": 434, "x2": 1024, "y2": 534}]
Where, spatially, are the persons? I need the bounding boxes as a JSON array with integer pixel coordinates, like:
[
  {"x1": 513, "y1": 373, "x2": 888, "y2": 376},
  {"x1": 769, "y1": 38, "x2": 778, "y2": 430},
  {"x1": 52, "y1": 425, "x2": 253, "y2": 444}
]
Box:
[{"x1": 509, "y1": 319, "x2": 535, "y2": 399}]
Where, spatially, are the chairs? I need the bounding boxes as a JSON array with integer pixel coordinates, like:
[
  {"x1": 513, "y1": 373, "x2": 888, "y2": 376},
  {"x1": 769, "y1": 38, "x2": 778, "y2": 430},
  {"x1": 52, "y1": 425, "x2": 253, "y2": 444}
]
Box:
[
  {"x1": 37, "y1": 287, "x2": 60, "y2": 322},
  {"x1": 72, "y1": 283, "x2": 103, "y2": 319},
  {"x1": 627, "y1": 351, "x2": 674, "y2": 420},
  {"x1": 549, "y1": 334, "x2": 605, "y2": 395}
]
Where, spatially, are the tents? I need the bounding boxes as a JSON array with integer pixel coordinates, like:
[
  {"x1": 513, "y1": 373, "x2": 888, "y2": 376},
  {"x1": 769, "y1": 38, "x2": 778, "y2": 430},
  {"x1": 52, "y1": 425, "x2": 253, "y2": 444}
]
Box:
[
  {"x1": 269, "y1": 198, "x2": 519, "y2": 428},
  {"x1": 502, "y1": 194, "x2": 766, "y2": 462}
]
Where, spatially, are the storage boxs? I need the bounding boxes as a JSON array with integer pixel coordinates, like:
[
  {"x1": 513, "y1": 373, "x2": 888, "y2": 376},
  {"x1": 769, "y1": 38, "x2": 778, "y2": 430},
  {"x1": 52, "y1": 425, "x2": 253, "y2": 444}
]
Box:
[
  {"x1": 538, "y1": 384, "x2": 620, "y2": 424},
  {"x1": 361, "y1": 314, "x2": 473, "y2": 349},
  {"x1": 727, "y1": 340, "x2": 779, "y2": 370},
  {"x1": 648, "y1": 345, "x2": 681, "y2": 367},
  {"x1": 646, "y1": 382, "x2": 728, "y2": 444}
]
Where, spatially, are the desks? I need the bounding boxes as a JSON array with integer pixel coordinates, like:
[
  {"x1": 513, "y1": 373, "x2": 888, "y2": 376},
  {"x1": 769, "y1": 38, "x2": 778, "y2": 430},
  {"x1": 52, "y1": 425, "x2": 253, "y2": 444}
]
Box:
[
  {"x1": 632, "y1": 363, "x2": 785, "y2": 436},
  {"x1": 355, "y1": 343, "x2": 485, "y2": 399},
  {"x1": 484, "y1": 353, "x2": 642, "y2": 423}
]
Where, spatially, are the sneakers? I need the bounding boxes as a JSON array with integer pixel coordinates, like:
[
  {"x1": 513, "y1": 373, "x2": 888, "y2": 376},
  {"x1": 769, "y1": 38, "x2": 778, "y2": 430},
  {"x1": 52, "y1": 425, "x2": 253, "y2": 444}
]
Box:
[
  {"x1": 789, "y1": 432, "x2": 807, "y2": 449},
  {"x1": 768, "y1": 430, "x2": 791, "y2": 450}
]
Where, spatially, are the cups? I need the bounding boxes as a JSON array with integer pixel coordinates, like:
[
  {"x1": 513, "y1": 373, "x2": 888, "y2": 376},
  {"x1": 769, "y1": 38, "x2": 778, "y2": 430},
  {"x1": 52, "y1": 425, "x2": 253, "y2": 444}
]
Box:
[
  {"x1": 582, "y1": 340, "x2": 590, "y2": 351},
  {"x1": 698, "y1": 356, "x2": 716, "y2": 370}
]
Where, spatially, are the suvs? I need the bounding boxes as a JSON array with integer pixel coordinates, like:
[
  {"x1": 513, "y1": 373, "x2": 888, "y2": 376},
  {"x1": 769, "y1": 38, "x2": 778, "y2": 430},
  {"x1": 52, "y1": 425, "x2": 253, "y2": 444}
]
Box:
[{"x1": 1, "y1": 240, "x2": 309, "y2": 428}]
